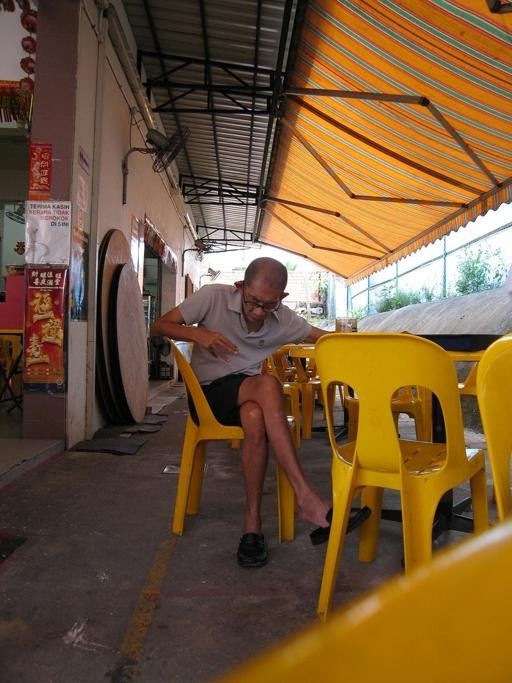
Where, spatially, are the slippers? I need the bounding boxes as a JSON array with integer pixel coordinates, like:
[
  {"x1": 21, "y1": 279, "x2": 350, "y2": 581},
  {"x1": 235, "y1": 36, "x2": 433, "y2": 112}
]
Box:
[
  {"x1": 310, "y1": 507, "x2": 370, "y2": 545},
  {"x1": 236, "y1": 533, "x2": 271, "y2": 569}
]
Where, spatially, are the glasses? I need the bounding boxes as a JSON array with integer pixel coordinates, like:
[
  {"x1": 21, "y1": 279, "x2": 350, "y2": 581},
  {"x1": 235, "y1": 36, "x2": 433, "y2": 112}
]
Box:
[{"x1": 241, "y1": 280, "x2": 283, "y2": 313}]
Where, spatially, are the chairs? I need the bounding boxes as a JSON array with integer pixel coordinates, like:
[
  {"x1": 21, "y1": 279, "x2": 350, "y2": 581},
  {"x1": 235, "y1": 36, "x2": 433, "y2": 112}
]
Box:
[
  {"x1": 341, "y1": 381, "x2": 423, "y2": 444},
  {"x1": 265, "y1": 342, "x2": 336, "y2": 448},
  {"x1": 164, "y1": 336, "x2": 298, "y2": 543},
  {"x1": 214, "y1": 519, "x2": 509, "y2": 681},
  {"x1": 480, "y1": 335, "x2": 509, "y2": 526},
  {"x1": 313, "y1": 333, "x2": 491, "y2": 623},
  {"x1": 435, "y1": 347, "x2": 483, "y2": 396}
]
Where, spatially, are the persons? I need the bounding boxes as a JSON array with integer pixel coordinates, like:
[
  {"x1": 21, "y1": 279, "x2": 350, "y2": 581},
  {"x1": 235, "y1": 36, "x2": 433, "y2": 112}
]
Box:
[{"x1": 149, "y1": 256, "x2": 413, "y2": 568}]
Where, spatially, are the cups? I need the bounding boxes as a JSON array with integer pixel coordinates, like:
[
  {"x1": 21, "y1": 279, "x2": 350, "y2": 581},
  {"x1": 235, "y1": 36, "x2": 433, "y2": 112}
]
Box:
[{"x1": 334, "y1": 315, "x2": 358, "y2": 331}]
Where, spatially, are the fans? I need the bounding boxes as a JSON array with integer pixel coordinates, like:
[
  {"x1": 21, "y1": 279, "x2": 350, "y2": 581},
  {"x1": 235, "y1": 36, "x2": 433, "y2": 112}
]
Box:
[
  {"x1": 199, "y1": 266, "x2": 222, "y2": 289},
  {"x1": 181, "y1": 246, "x2": 207, "y2": 276},
  {"x1": 4, "y1": 204, "x2": 26, "y2": 225},
  {"x1": 121, "y1": 122, "x2": 194, "y2": 203}
]
